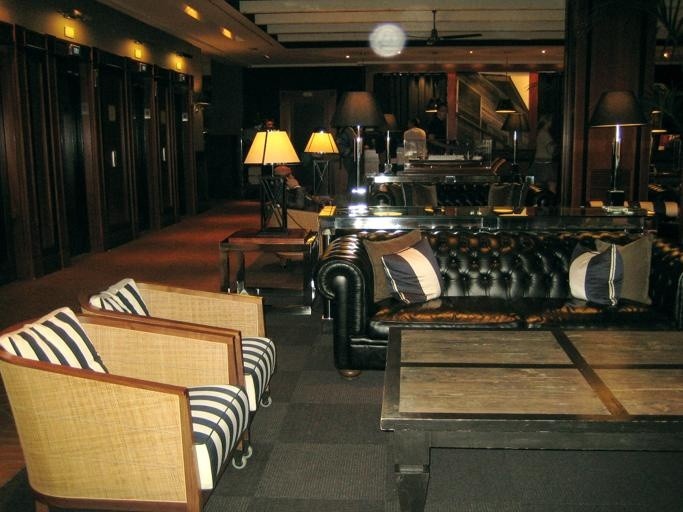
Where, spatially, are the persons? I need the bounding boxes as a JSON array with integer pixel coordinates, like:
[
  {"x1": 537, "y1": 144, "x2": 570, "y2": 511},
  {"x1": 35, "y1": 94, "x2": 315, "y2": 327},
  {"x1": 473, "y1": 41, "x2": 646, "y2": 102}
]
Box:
[
  {"x1": 429, "y1": 104, "x2": 448, "y2": 155},
  {"x1": 273, "y1": 167, "x2": 323, "y2": 213},
  {"x1": 404, "y1": 118, "x2": 427, "y2": 157},
  {"x1": 529, "y1": 114, "x2": 559, "y2": 193}
]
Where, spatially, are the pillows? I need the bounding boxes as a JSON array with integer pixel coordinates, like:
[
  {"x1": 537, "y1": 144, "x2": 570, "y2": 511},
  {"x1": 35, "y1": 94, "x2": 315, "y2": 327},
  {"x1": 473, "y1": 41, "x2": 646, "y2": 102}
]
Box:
[
  {"x1": 488, "y1": 182, "x2": 527, "y2": 207},
  {"x1": 380, "y1": 237, "x2": 444, "y2": 305},
  {"x1": 410, "y1": 182, "x2": 437, "y2": 207},
  {"x1": 593, "y1": 233, "x2": 654, "y2": 305},
  {"x1": 361, "y1": 228, "x2": 422, "y2": 304},
  {"x1": 568, "y1": 243, "x2": 624, "y2": 308}
]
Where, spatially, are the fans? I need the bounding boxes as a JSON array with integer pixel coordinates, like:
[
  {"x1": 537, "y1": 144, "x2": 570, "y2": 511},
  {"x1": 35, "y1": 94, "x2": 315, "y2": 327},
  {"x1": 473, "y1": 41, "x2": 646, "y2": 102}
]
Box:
[{"x1": 405, "y1": 11, "x2": 483, "y2": 45}]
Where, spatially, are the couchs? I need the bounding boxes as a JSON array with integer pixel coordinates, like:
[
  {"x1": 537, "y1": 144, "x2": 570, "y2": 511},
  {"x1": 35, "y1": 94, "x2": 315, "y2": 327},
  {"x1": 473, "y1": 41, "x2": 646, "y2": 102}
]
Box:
[
  {"x1": 0, "y1": 306, "x2": 250, "y2": 512},
  {"x1": 76, "y1": 276, "x2": 277, "y2": 459},
  {"x1": 313, "y1": 228, "x2": 682, "y2": 381},
  {"x1": 368, "y1": 180, "x2": 559, "y2": 215}
]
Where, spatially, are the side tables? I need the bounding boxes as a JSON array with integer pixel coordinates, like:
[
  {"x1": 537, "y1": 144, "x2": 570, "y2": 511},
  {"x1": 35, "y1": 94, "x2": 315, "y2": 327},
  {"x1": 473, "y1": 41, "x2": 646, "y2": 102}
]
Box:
[{"x1": 220, "y1": 227, "x2": 318, "y2": 307}]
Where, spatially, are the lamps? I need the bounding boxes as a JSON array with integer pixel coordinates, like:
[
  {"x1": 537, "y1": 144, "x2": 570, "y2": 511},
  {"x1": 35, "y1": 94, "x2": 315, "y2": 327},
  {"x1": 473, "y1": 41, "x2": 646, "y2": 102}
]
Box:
[
  {"x1": 242, "y1": 129, "x2": 300, "y2": 235},
  {"x1": 303, "y1": 130, "x2": 339, "y2": 198},
  {"x1": 493, "y1": 55, "x2": 516, "y2": 114},
  {"x1": 329, "y1": 89, "x2": 390, "y2": 208},
  {"x1": 423, "y1": 71, "x2": 444, "y2": 114},
  {"x1": 588, "y1": 89, "x2": 646, "y2": 207},
  {"x1": 500, "y1": 112, "x2": 531, "y2": 174},
  {"x1": 647, "y1": 109, "x2": 669, "y2": 186}
]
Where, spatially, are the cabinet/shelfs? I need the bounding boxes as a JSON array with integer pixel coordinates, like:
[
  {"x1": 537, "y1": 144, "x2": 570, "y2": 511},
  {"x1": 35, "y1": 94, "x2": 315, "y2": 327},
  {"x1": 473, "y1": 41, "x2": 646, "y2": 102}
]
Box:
[{"x1": 0, "y1": 19, "x2": 200, "y2": 289}]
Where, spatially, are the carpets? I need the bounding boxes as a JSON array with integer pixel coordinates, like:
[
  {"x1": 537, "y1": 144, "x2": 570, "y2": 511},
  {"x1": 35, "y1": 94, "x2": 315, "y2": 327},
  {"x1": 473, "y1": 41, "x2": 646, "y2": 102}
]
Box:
[{"x1": 4, "y1": 251, "x2": 683, "y2": 512}]
